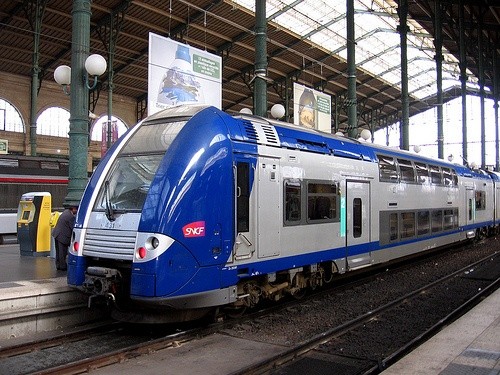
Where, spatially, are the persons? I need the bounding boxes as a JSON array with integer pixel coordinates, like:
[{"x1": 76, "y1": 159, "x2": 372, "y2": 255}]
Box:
[{"x1": 51, "y1": 206, "x2": 79, "y2": 271}]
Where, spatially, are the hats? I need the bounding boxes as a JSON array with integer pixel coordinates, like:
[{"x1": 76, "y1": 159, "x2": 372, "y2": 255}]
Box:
[{"x1": 175, "y1": 45, "x2": 191, "y2": 63}]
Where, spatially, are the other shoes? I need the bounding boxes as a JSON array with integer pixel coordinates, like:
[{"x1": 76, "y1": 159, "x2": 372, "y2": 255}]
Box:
[{"x1": 57, "y1": 265, "x2": 69, "y2": 271}]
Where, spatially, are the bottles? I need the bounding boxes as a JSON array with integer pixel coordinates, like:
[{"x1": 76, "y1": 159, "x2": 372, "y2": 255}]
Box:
[
  {"x1": 299, "y1": 88, "x2": 318, "y2": 130},
  {"x1": 156, "y1": 43, "x2": 204, "y2": 113}
]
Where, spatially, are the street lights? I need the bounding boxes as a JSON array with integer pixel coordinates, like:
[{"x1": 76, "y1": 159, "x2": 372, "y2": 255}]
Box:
[{"x1": 51, "y1": 54, "x2": 109, "y2": 213}]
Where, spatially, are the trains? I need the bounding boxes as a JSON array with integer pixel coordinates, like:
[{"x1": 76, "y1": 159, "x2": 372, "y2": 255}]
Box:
[
  {"x1": 66, "y1": 105, "x2": 499, "y2": 333},
  {"x1": 0, "y1": 154, "x2": 103, "y2": 247}
]
